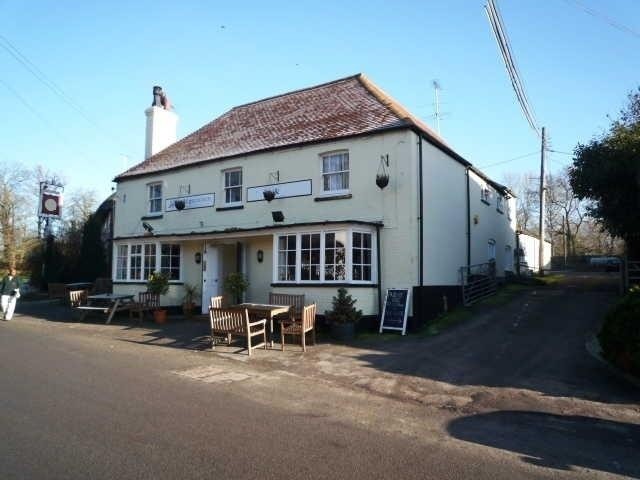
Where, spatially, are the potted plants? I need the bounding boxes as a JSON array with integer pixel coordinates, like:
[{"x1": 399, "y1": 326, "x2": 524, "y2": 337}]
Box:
[
  {"x1": 177, "y1": 278, "x2": 204, "y2": 315},
  {"x1": 322, "y1": 286, "x2": 365, "y2": 342},
  {"x1": 144, "y1": 267, "x2": 176, "y2": 326}
]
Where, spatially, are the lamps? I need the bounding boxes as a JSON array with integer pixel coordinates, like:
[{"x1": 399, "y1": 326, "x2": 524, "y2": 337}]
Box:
[
  {"x1": 141, "y1": 220, "x2": 154, "y2": 237},
  {"x1": 256, "y1": 249, "x2": 265, "y2": 263},
  {"x1": 194, "y1": 252, "x2": 201, "y2": 265},
  {"x1": 271, "y1": 211, "x2": 287, "y2": 228}
]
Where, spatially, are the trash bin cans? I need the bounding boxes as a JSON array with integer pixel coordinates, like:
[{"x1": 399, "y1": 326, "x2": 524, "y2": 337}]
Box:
[{"x1": 65, "y1": 285, "x2": 88, "y2": 304}]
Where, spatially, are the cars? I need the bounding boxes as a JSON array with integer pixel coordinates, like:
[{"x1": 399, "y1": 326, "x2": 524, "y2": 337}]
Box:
[
  {"x1": 590, "y1": 254, "x2": 605, "y2": 269},
  {"x1": 604, "y1": 255, "x2": 624, "y2": 269},
  {"x1": 17, "y1": 275, "x2": 41, "y2": 298}
]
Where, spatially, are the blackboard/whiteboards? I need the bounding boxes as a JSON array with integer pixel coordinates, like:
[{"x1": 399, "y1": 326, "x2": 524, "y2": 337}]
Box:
[{"x1": 380, "y1": 289, "x2": 410, "y2": 330}]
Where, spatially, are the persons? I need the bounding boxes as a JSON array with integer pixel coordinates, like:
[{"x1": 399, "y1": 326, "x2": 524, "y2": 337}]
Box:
[{"x1": 0, "y1": 266, "x2": 21, "y2": 320}]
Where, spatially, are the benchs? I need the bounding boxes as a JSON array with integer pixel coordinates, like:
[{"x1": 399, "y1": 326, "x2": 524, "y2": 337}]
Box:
[
  {"x1": 68, "y1": 288, "x2": 157, "y2": 328},
  {"x1": 205, "y1": 290, "x2": 319, "y2": 357}
]
